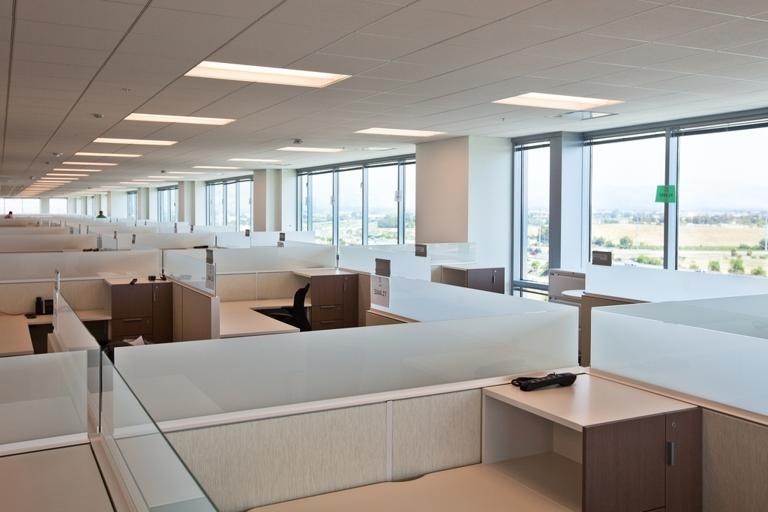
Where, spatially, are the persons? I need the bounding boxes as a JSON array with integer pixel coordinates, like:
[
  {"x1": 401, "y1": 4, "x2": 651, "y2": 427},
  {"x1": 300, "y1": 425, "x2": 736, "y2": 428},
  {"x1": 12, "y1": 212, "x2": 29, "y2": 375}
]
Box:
[
  {"x1": 96, "y1": 210, "x2": 106, "y2": 218},
  {"x1": 5, "y1": 212, "x2": 12, "y2": 219}
]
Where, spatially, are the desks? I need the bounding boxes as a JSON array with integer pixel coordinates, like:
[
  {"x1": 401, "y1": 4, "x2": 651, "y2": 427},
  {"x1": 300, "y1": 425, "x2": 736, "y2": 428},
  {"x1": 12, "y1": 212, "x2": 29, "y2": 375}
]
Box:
[
  {"x1": 0, "y1": 310, "x2": 112, "y2": 358},
  {"x1": 241, "y1": 451, "x2": 583, "y2": 512},
  {"x1": 219, "y1": 299, "x2": 314, "y2": 338}
]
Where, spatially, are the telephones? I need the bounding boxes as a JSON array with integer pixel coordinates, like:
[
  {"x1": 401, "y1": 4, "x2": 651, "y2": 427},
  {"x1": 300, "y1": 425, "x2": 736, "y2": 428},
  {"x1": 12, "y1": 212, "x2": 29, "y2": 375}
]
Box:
[{"x1": 35, "y1": 297, "x2": 53, "y2": 315}]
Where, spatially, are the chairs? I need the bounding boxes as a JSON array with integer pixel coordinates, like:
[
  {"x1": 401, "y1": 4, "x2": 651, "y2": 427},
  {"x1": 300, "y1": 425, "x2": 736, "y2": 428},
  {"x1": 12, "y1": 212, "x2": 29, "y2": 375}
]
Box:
[{"x1": 267, "y1": 283, "x2": 313, "y2": 328}]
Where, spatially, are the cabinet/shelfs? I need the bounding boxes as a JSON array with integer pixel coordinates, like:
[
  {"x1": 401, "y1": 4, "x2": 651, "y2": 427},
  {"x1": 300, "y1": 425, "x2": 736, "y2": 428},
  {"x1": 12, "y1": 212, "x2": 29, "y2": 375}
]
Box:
[
  {"x1": 104, "y1": 275, "x2": 174, "y2": 344},
  {"x1": 476, "y1": 369, "x2": 704, "y2": 509},
  {"x1": 445, "y1": 263, "x2": 506, "y2": 294},
  {"x1": 292, "y1": 265, "x2": 360, "y2": 329}
]
